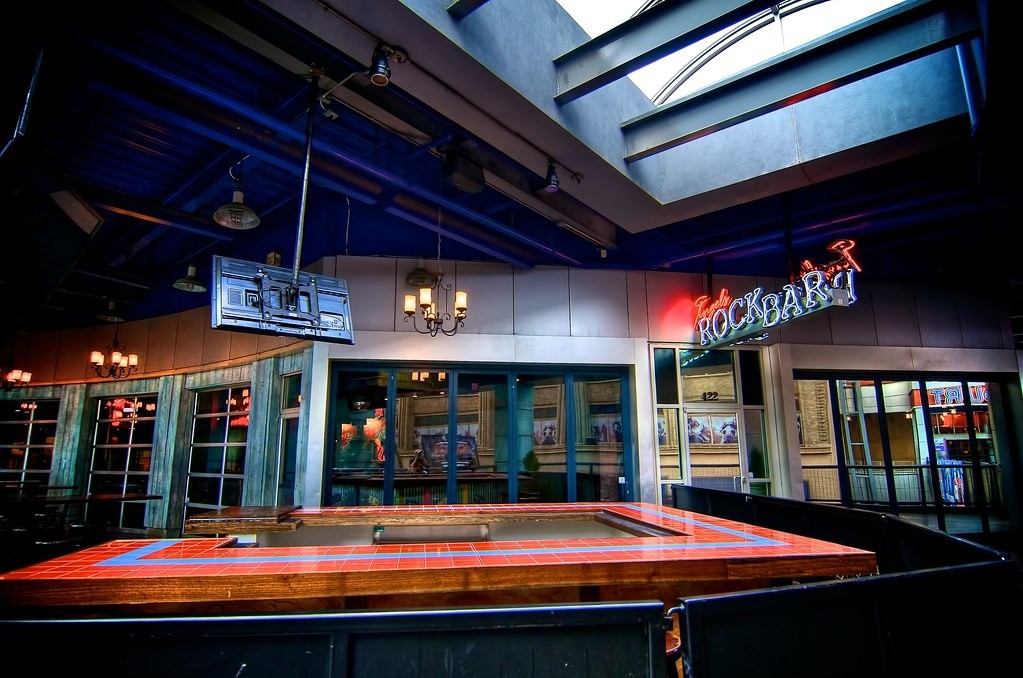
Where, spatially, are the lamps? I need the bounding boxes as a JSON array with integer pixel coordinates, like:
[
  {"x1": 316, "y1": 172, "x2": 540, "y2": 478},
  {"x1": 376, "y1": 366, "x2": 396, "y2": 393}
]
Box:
[
  {"x1": 97, "y1": 297, "x2": 125, "y2": 323},
  {"x1": 370, "y1": 44, "x2": 391, "y2": 87},
  {"x1": 404, "y1": 287, "x2": 467, "y2": 338},
  {"x1": 89, "y1": 323, "x2": 138, "y2": 380},
  {"x1": 544, "y1": 160, "x2": 559, "y2": 192},
  {"x1": 172, "y1": 264, "x2": 207, "y2": 293},
  {"x1": 0, "y1": 368, "x2": 32, "y2": 393},
  {"x1": 446, "y1": 155, "x2": 484, "y2": 194},
  {"x1": 213, "y1": 153, "x2": 260, "y2": 229}
]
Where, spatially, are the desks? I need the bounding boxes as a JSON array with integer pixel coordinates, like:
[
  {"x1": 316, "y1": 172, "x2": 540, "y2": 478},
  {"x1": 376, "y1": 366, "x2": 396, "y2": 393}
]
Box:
[{"x1": 0, "y1": 503, "x2": 880, "y2": 678}]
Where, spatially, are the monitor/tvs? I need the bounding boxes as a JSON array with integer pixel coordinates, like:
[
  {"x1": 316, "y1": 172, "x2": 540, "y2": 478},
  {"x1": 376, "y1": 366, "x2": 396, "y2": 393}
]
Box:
[{"x1": 211, "y1": 255, "x2": 355, "y2": 345}]
